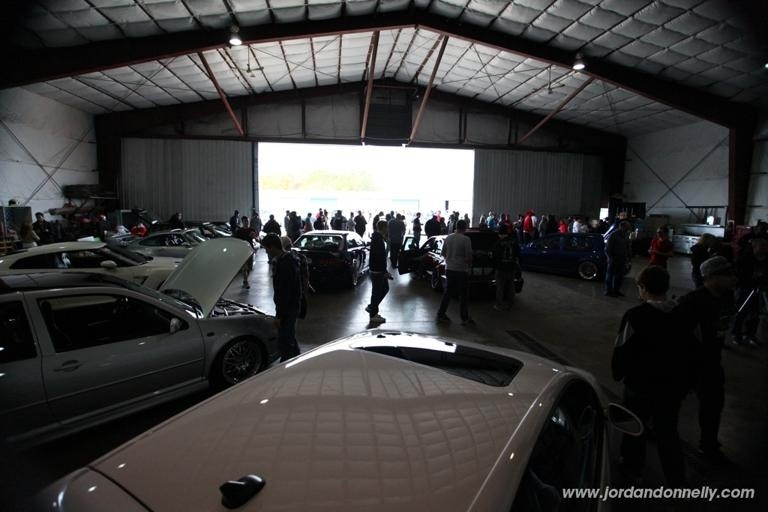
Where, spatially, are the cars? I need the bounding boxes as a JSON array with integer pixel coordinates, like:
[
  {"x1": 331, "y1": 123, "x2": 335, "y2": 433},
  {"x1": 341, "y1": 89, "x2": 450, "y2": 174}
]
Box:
[
  {"x1": 271, "y1": 228, "x2": 378, "y2": 291},
  {"x1": 30, "y1": 328, "x2": 650, "y2": 512},
  {"x1": 0, "y1": 235, "x2": 283, "y2": 459},
  {"x1": 0, "y1": 206, "x2": 269, "y2": 306},
  {"x1": 521, "y1": 219, "x2": 646, "y2": 283},
  {"x1": 396, "y1": 234, "x2": 525, "y2": 300}
]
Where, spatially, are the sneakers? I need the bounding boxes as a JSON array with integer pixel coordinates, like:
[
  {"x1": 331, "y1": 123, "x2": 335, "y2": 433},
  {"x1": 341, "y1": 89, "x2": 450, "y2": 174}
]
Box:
[{"x1": 366, "y1": 305, "x2": 385, "y2": 323}]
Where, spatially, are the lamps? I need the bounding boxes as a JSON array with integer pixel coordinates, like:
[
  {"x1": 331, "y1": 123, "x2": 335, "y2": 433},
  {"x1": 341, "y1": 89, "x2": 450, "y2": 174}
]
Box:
[
  {"x1": 225, "y1": 24, "x2": 244, "y2": 47},
  {"x1": 570, "y1": 52, "x2": 584, "y2": 73}
]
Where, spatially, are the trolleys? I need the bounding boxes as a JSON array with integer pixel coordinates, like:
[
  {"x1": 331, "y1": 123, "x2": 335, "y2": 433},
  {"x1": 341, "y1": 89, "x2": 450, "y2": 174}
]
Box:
[{"x1": 726, "y1": 287, "x2": 759, "y2": 348}]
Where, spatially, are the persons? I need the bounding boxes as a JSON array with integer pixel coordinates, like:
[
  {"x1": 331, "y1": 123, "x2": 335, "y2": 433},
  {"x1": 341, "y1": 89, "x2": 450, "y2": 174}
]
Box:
[
  {"x1": 258, "y1": 231, "x2": 301, "y2": 363},
  {"x1": 688, "y1": 216, "x2": 767, "y2": 351},
  {"x1": 279, "y1": 236, "x2": 316, "y2": 320},
  {"x1": 488, "y1": 227, "x2": 515, "y2": 312},
  {"x1": 673, "y1": 256, "x2": 740, "y2": 473},
  {"x1": 363, "y1": 220, "x2": 395, "y2": 324},
  {"x1": 0, "y1": 193, "x2": 674, "y2": 298},
  {"x1": 434, "y1": 220, "x2": 479, "y2": 328},
  {"x1": 609, "y1": 262, "x2": 701, "y2": 482}
]
